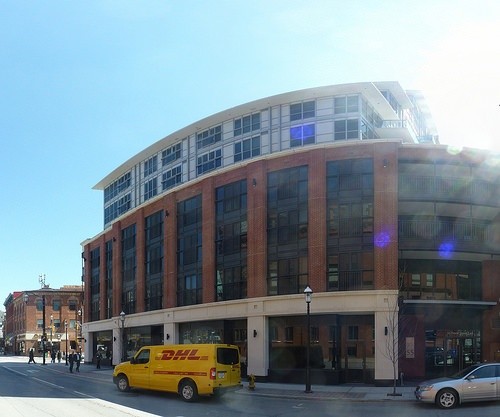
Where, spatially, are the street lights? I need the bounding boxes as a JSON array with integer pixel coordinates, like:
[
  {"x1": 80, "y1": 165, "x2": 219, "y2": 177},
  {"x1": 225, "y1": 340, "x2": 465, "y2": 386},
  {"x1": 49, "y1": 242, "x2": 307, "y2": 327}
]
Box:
[
  {"x1": 50, "y1": 314, "x2": 54, "y2": 343},
  {"x1": 303, "y1": 286, "x2": 313, "y2": 394},
  {"x1": 120, "y1": 311, "x2": 126, "y2": 363},
  {"x1": 64, "y1": 320, "x2": 69, "y2": 366},
  {"x1": 78, "y1": 309, "x2": 82, "y2": 356}
]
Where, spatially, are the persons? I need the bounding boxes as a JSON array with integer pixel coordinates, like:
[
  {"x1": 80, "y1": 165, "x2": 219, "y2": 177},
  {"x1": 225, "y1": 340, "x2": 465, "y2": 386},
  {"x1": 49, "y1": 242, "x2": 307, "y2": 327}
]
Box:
[
  {"x1": 74, "y1": 350, "x2": 82, "y2": 373},
  {"x1": 96, "y1": 349, "x2": 103, "y2": 369},
  {"x1": 57, "y1": 351, "x2": 62, "y2": 363},
  {"x1": 27, "y1": 349, "x2": 36, "y2": 364},
  {"x1": 51, "y1": 350, "x2": 56, "y2": 363},
  {"x1": 68, "y1": 350, "x2": 75, "y2": 373}
]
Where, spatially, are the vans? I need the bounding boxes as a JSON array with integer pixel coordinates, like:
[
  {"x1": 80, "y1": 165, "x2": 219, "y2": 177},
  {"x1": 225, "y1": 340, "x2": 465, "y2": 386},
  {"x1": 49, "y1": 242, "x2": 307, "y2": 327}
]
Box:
[{"x1": 112, "y1": 343, "x2": 243, "y2": 402}]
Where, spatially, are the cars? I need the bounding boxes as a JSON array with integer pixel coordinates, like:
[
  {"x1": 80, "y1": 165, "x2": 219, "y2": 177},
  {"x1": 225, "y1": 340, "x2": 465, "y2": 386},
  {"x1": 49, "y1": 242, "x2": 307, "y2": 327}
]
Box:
[{"x1": 414, "y1": 361, "x2": 500, "y2": 410}]
